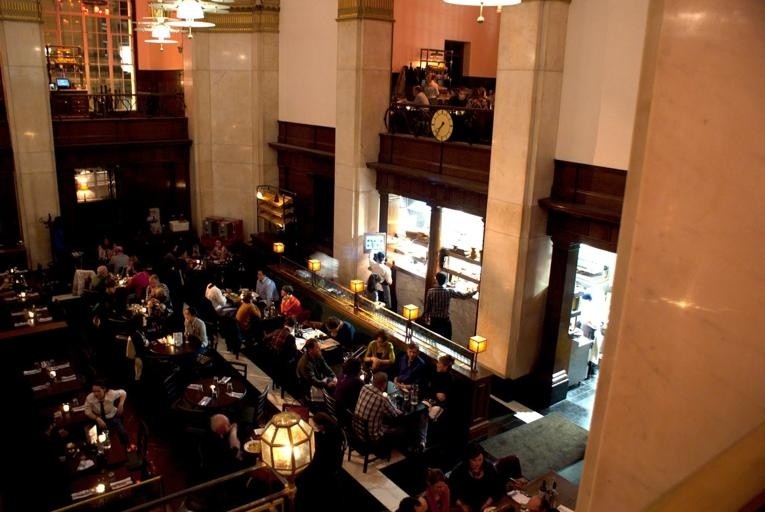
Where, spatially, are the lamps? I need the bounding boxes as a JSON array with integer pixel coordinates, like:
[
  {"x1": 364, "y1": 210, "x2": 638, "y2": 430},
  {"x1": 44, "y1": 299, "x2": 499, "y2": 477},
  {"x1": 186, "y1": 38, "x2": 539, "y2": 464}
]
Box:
[
  {"x1": 133, "y1": 0, "x2": 180, "y2": 52},
  {"x1": 273, "y1": 241, "x2": 286, "y2": 268},
  {"x1": 163, "y1": 0, "x2": 234, "y2": 38}
]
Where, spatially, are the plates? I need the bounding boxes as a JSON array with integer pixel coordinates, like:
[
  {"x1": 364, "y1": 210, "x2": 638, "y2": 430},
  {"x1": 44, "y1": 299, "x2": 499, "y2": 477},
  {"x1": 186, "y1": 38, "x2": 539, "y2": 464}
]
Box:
[{"x1": 243, "y1": 439, "x2": 262, "y2": 454}]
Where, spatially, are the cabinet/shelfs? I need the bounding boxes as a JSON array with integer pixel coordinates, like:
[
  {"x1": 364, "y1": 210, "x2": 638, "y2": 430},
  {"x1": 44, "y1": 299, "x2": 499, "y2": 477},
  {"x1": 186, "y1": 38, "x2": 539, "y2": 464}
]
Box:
[
  {"x1": 253, "y1": 184, "x2": 297, "y2": 233},
  {"x1": 392, "y1": 232, "x2": 481, "y2": 288},
  {"x1": 419, "y1": 48, "x2": 454, "y2": 89},
  {"x1": 570, "y1": 289, "x2": 584, "y2": 319},
  {"x1": 45, "y1": 44, "x2": 85, "y2": 88}
]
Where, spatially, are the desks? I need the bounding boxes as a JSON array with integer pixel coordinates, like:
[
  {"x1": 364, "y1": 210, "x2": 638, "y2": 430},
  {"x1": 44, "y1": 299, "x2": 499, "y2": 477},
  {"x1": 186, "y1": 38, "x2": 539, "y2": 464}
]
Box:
[{"x1": 49, "y1": 90, "x2": 88, "y2": 118}]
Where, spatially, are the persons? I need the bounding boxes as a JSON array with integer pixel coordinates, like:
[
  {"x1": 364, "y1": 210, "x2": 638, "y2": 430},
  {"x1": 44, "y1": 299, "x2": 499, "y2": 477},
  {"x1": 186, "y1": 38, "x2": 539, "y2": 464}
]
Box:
[{"x1": 410, "y1": 72, "x2": 495, "y2": 111}]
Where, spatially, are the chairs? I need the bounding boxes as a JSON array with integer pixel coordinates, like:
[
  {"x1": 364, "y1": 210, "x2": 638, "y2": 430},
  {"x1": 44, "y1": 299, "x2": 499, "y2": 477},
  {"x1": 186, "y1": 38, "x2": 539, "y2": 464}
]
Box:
[{"x1": 3, "y1": 251, "x2": 523, "y2": 511}]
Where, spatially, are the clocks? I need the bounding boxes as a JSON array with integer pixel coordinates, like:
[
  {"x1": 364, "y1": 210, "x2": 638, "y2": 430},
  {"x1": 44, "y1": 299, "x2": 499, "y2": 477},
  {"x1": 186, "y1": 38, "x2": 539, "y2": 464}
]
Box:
[{"x1": 429, "y1": 108, "x2": 455, "y2": 142}]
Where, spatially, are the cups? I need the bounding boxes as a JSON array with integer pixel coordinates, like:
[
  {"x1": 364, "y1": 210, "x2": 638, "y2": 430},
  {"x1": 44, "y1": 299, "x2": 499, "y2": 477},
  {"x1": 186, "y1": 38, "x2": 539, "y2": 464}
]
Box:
[
  {"x1": 212, "y1": 391, "x2": 217, "y2": 398},
  {"x1": 264, "y1": 309, "x2": 270, "y2": 318},
  {"x1": 212, "y1": 376, "x2": 219, "y2": 384},
  {"x1": 198, "y1": 384, "x2": 204, "y2": 392},
  {"x1": 227, "y1": 382, "x2": 234, "y2": 394},
  {"x1": 132, "y1": 303, "x2": 144, "y2": 314},
  {"x1": 65, "y1": 443, "x2": 76, "y2": 453}
]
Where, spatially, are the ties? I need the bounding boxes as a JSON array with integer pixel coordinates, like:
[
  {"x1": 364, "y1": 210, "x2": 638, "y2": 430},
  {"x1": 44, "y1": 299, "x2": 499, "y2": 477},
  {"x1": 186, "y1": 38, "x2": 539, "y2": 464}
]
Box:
[{"x1": 98, "y1": 401, "x2": 106, "y2": 422}]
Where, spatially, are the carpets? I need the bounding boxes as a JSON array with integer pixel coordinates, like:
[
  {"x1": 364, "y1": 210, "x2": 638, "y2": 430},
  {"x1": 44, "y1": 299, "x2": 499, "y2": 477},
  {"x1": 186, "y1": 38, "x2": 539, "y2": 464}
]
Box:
[{"x1": 479, "y1": 411, "x2": 589, "y2": 483}]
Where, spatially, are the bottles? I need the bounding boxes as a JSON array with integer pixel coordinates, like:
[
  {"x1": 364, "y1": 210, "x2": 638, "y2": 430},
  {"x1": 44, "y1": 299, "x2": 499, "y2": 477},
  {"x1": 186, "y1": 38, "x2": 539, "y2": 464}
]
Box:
[
  {"x1": 538, "y1": 479, "x2": 559, "y2": 510},
  {"x1": 393, "y1": 384, "x2": 420, "y2": 406},
  {"x1": 295, "y1": 321, "x2": 304, "y2": 338},
  {"x1": 270, "y1": 302, "x2": 277, "y2": 317},
  {"x1": 98, "y1": 430, "x2": 112, "y2": 454}
]
